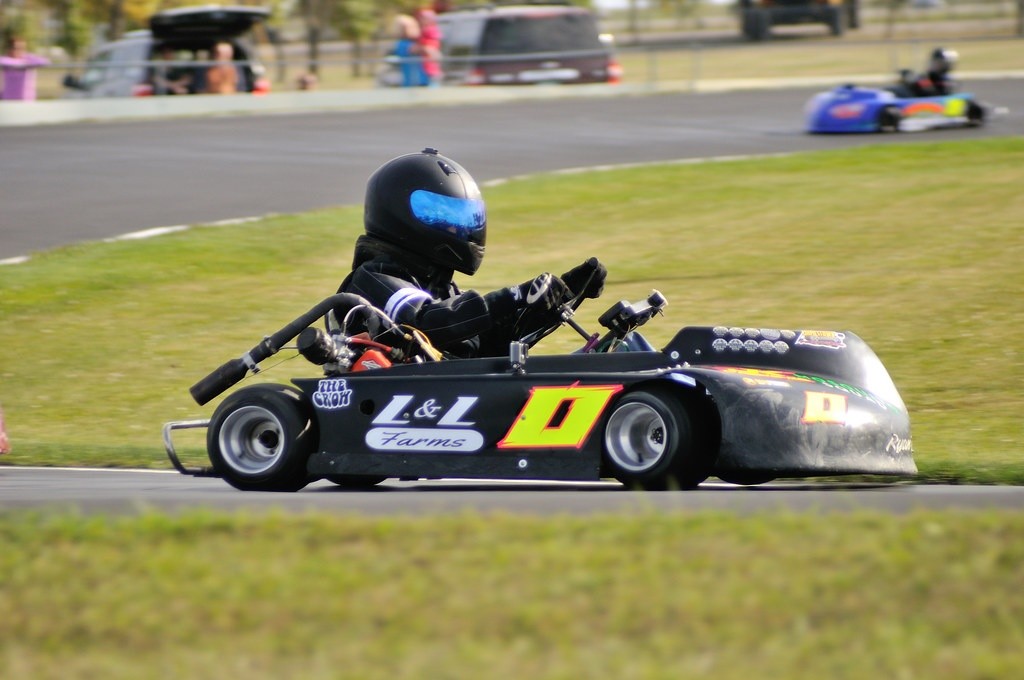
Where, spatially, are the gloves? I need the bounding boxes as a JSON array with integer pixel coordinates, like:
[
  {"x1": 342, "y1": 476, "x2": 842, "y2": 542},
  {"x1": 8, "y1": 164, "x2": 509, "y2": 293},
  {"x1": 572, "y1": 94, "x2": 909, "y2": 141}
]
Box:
[{"x1": 559, "y1": 257, "x2": 608, "y2": 299}]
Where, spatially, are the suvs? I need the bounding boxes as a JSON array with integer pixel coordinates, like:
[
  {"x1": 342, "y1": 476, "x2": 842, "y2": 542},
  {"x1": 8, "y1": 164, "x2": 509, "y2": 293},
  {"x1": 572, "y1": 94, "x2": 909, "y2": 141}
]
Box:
[
  {"x1": 386, "y1": 5, "x2": 621, "y2": 86},
  {"x1": 64, "y1": 6, "x2": 275, "y2": 98},
  {"x1": 732, "y1": 0, "x2": 860, "y2": 38}
]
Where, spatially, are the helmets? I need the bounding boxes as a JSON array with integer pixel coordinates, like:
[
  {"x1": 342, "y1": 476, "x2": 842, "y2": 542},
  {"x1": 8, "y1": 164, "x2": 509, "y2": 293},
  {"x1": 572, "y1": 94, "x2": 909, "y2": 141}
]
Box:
[
  {"x1": 363, "y1": 147, "x2": 487, "y2": 277},
  {"x1": 930, "y1": 49, "x2": 958, "y2": 68}
]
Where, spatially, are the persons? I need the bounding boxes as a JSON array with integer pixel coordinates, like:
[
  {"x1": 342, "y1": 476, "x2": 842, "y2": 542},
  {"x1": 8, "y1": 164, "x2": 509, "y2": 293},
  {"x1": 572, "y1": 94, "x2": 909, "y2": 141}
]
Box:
[
  {"x1": 884, "y1": 47, "x2": 960, "y2": 100},
  {"x1": 154, "y1": 43, "x2": 245, "y2": 97},
  {"x1": 0, "y1": 37, "x2": 49, "y2": 100},
  {"x1": 324, "y1": 147, "x2": 607, "y2": 360},
  {"x1": 390, "y1": 9, "x2": 442, "y2": 88}
]
